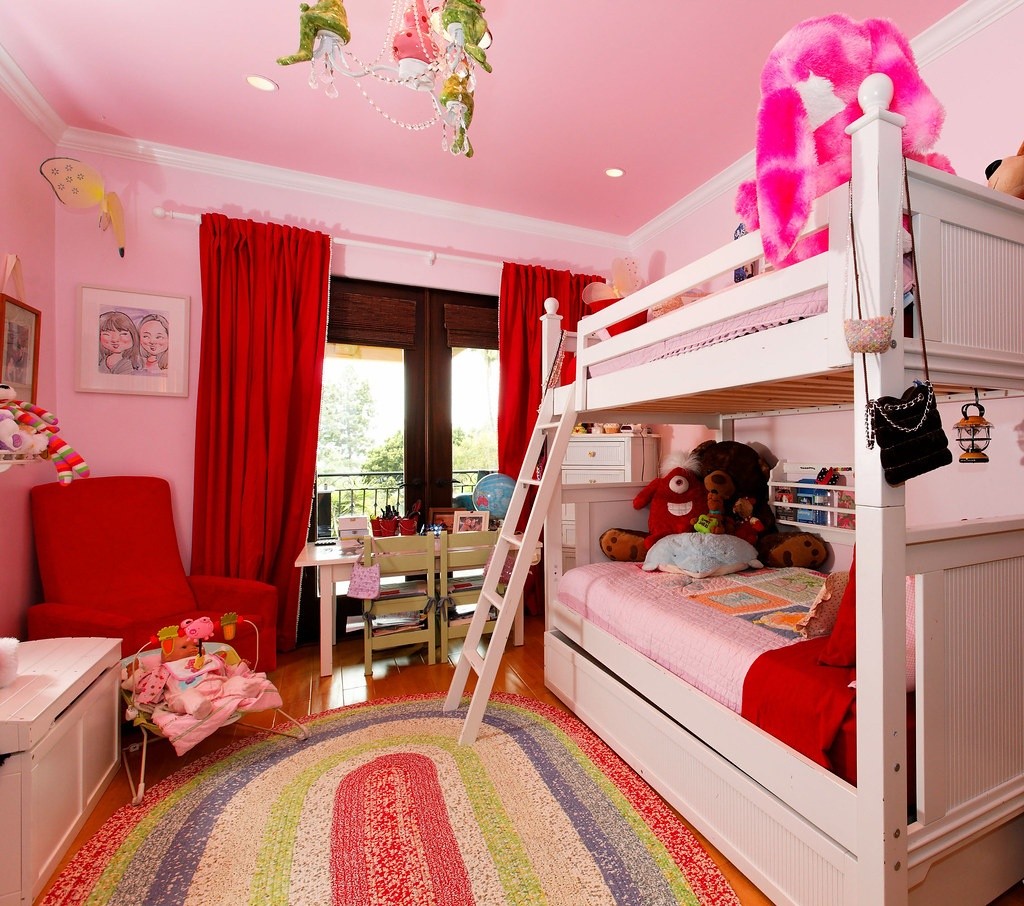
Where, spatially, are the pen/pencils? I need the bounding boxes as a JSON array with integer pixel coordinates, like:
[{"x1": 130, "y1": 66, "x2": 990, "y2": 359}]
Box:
[{"x1": 381, "y1": 505, "x2": 400, "y2": 519}]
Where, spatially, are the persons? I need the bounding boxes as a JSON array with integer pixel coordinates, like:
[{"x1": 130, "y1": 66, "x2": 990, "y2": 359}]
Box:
[
  {"x1": 121, "y1": 628, "x2": 259, "y2": 720},
  {"x1": 460, "y1": 519, "x2": 482, "y2": 531}
]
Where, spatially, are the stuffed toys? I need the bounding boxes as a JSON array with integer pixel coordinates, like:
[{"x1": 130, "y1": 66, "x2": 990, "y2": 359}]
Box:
[
  {"x1": 599, "y1": 440, "x2": 828, "y2": 569},
  {"x1": 736, "y1": 13, "x2": 957, "y2": 264},
  {"x1": 985, "y1": 141, "x2": 1024, "y2": 201},
  {"x1": 0, "y1": 385, "x2": 90, "y2": 487}
]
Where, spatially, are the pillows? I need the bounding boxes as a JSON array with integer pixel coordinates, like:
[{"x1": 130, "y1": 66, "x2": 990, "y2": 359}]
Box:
[
  {"x1": 792, "y1": 573, "x2": 852, "y2": 637},
  {"x1": 846, "y1": 573, "x2": 919, "y2": 695}
]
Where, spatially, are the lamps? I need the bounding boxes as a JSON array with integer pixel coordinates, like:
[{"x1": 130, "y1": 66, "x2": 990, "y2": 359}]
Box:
[
  {"x1": 952, "y1": 402, "x2": 993, "y2": 463},
  {"x1": 277, "y1": 0, "x2": 493, "y2": 157}
]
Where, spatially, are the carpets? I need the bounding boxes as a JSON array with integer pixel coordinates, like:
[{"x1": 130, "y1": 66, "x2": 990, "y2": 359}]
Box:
[{"x1": 43, "y1": 691, "x2": 742, "y2": 906}]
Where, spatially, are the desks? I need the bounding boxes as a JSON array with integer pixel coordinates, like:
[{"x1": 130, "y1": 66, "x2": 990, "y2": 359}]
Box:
[{"x1": 294, "y1": 533, "x2": 544, "y2": 678}]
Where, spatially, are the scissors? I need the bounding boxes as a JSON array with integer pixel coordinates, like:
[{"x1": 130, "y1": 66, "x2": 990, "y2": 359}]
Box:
[{"x1": 413, "y1": 500, "x2": 422, "y2": 511}]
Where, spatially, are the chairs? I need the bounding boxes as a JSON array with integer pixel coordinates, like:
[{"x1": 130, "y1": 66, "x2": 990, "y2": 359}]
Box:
[
  {"x1": 361, "y1": 531, "x2": 436, "y2": 674},
  {"x1": 436, "y1": 528, "x2": 507, "y2": 662}
]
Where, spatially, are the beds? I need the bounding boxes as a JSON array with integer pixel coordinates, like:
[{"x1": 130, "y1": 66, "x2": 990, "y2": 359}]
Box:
[{"x1": 538, "y1": 70, "x2": 1024, "y2": 906}]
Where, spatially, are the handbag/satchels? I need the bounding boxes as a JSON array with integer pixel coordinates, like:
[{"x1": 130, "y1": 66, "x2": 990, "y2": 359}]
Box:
[
  {"x1": 864, "y1": 378, "x2": 952, "y2": 487},
  {"x1": 346, "y1": 537, "x2": 380, "y2": 600},
  {"x1": 843, "y1": 316, "x2": 893, "y2": 353}
]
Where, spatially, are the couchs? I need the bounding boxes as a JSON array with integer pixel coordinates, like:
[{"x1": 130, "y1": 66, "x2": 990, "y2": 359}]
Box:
[{"x1": 26, "y1": 476, "x2": 279, "y2": 673}]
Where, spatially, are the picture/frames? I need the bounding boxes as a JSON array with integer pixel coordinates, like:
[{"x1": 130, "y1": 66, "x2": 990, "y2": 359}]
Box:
[
  {"x1": 429, "y1": 507, "x2": 467, "y2": 534},
  {"x1": 0, "y1": 292, "x2": 42, "y2": 407},
  {"x1": 453, "y1": 510, "x2": 490, "y2": 533},
  {"x1": 74, "y1": 286, "x2": 192, "y2": 398}
]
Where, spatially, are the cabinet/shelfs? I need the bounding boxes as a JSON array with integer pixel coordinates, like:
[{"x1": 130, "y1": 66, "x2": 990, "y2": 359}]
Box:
[
  {"x1": 768, "y1": 459, "x2": 857, "y2": 535},
  {"x1": 0, "y1": 635, "x2": 123, "y2": 906},
  {"x1": 560, "y1": 431, "x2": 661, "y2": 576}
]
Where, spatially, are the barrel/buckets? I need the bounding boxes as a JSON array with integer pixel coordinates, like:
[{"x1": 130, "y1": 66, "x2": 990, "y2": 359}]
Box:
[{"x1": 371, "y1": 515, "x2": 418, "y2": 537}]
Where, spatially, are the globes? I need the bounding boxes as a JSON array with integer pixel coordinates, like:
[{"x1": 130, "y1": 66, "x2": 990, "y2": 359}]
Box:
[{"x1": 473, "y1": 472, "x2": 516, "y2": 523}]
